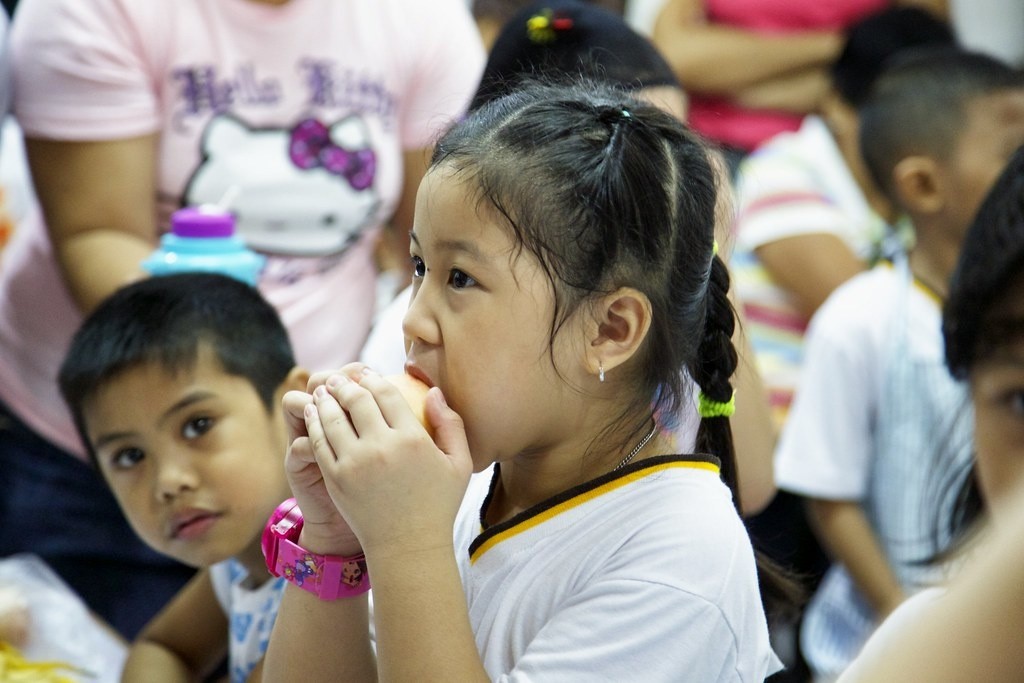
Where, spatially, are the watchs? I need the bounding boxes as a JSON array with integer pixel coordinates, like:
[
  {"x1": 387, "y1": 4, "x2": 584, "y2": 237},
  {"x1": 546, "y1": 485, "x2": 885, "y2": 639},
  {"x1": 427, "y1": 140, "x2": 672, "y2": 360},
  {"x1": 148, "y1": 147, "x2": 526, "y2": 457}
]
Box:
[{"x1": 261, "y1": 497, "x2": 370, "y2": 600}]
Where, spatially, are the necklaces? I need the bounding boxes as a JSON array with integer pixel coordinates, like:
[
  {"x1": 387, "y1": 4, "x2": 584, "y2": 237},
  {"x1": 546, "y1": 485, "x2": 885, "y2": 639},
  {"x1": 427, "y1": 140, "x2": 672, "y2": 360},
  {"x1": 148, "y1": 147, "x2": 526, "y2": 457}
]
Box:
[{"x1": 612, "y1": 417, "x2": 657, "y2": 471}]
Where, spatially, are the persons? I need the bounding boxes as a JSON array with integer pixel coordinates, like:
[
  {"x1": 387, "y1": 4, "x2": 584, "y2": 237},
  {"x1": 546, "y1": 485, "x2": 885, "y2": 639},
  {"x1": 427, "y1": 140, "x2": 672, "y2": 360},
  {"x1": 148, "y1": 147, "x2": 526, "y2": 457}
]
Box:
[
  {"x1": 259, "y1": 91, "x2": 770, "y2": 683},
  {"x1": 469, "y1": 0, "x2": 777, "y2": 514},
  {"x1": 653, "y1": 0, "x2": 950, "y2": 181},
  {"x1": 57, "y1": 273, "x2": 378, "y2": 683},
  {"x1": 0, "y1": 0, "x2": 488, "y2": 683},
  {"x1": 837, "y1": 146, "x2": 1024, "y2": 683},
  {"x1": 773, "y1": 52, "x2": 1024, "y2": 683},
  {"x1": 728, "y1": 0, "x2": 966, "y2": 683}
]
